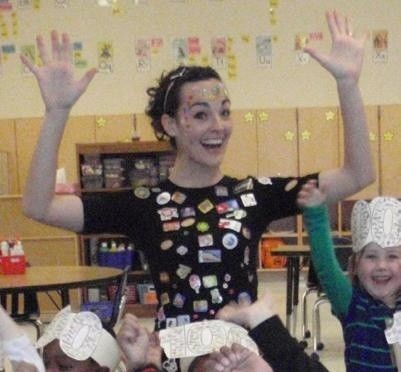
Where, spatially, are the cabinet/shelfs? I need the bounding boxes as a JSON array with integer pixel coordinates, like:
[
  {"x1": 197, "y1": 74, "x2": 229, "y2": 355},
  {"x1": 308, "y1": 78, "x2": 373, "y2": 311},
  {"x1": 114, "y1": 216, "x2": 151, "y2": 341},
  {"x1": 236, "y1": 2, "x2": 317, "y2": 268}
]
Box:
[
  {"x1": 254, "y1": 203, "x2": 356, "y2": 273},
  {"x1": 1, "y1": 103, "x2": 400, "y2": 203},
  {"x1": 0, "y1": 194, "x2": 83, "y2": 323},
  {"x1": 75, "y1": 141, "x2": 178, "y2": 317}
]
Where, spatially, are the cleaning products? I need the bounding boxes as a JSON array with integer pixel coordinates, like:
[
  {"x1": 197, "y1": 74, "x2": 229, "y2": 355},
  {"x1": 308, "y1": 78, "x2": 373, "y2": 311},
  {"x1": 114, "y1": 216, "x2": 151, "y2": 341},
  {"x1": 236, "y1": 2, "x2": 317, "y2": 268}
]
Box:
[{"x1": 10, "y1": 234, "x2": 25, "y2": 262}]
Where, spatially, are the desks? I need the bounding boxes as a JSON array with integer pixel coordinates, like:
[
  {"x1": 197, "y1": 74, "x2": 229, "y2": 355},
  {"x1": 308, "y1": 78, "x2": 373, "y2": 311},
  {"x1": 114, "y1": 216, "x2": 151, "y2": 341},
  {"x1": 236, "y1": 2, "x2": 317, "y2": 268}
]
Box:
[
  {"x1": 1, "y1": 265, "x2": 125, "y2": 339},
  {"x1": 270, "y1": 243, "x2": 353, "y2": 333}
]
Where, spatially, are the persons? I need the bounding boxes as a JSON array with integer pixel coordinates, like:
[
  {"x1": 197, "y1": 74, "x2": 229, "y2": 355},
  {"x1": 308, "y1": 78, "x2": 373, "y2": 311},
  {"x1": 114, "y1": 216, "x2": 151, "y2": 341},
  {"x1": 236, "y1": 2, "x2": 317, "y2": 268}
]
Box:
[
  {"x1": 19, "y1": 8, "x2": 382, "y2": 329},
  {"x1": 0, "y1": 287, "x2": 328, "y2": 372},
  {"x1": 298, "y1": 178, "x2": 401, "y2": 371}
]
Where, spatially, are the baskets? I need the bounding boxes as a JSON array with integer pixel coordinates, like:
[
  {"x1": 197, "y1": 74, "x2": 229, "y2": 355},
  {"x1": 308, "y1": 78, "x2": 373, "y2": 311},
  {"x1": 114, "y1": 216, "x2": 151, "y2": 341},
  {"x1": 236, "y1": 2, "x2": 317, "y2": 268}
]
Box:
[
  {"x1": 82, "y1": 299, "x2": 113, "y2": 325},
  {"x1": 109, "y1": 280, "x2": 159, "y2": 305},
  {"x1": 99, "y1": 249, "x2": 138, "y2": 269},
  {"x1": 0, "y1": 249, "x2": 26, "y2": 275}
]
